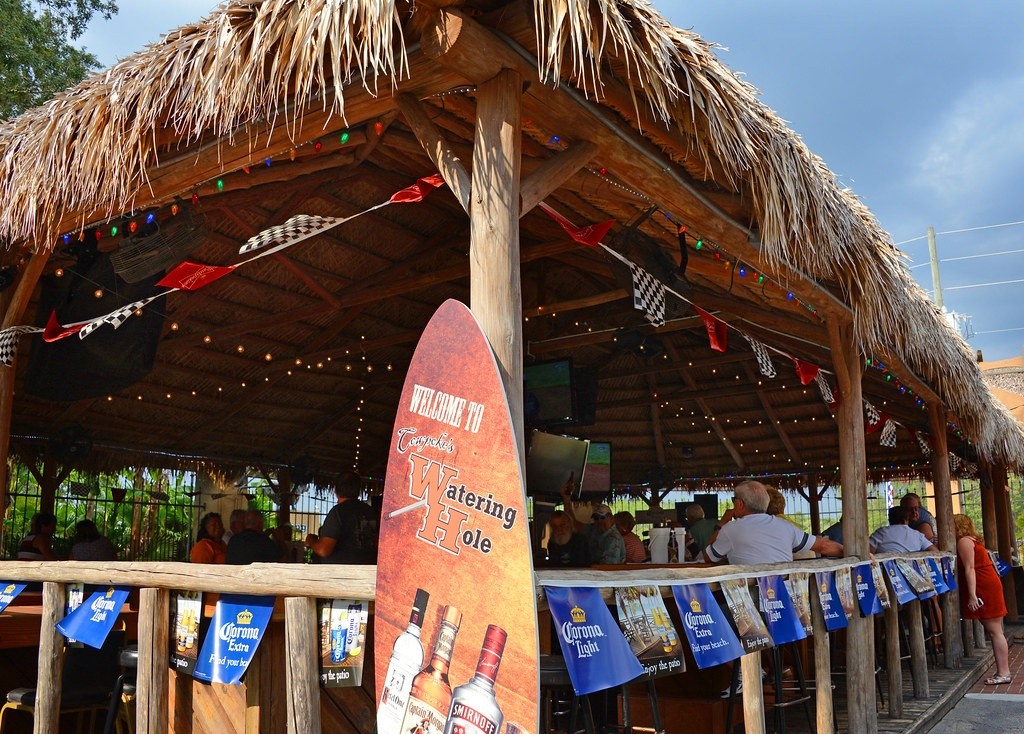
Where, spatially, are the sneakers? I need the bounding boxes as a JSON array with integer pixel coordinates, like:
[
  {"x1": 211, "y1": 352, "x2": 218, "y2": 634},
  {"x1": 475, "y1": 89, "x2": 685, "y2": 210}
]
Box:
[{"x1": 720, "y1": 668, "x2": 768, "y2": 699}]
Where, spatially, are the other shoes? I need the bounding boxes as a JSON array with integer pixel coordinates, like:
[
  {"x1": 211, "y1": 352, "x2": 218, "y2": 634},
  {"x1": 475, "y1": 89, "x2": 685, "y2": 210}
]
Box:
[{"x1": 985, "y1": 671, "x2": 1011, "y2": 685}]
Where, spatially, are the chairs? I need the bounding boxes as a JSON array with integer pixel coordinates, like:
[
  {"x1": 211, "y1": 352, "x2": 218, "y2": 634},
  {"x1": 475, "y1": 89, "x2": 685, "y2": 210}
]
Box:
[{"x1": 0, "y1": 629, "x2": 126, "y2": 734}]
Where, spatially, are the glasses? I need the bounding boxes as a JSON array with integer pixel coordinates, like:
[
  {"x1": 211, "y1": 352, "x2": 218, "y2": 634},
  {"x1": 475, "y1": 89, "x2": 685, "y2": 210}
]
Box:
[
  {"x1": 731, "y1": 497, "x2": 745, "y2": 504},
  {"x1": 685, "y1": 516, "x2": 688, "y2": 521},
  {"x1": 592, "y1": 513, "x2": 609, "y2": 520}
]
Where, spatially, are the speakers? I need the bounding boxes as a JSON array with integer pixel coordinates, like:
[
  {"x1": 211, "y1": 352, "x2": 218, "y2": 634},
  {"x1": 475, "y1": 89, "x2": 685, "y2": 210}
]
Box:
[
  {"x1": 675, "y1": 502, "x2": 696, "y2": 528},
  {"x1": 694, "y1": 494, "x2": 718, "y2": 520},
  {"x1": 25, "y1": 221, "x2": 166, "y2": 402}
]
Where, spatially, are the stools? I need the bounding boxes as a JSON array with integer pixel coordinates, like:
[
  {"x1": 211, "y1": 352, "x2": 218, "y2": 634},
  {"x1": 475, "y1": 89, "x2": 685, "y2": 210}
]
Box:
[
  {"x1": 539, "y1": 593, "x2": 946, "y2": 734},
  {"x1": 103, "y1": 646, "x2": 138, "y2": 734}
]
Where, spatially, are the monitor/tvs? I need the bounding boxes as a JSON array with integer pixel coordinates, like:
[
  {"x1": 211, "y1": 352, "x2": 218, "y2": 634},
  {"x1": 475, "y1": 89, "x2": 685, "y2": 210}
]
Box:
[{"x1": 521, "y1": 356, "x2": 612, "y2": 502}]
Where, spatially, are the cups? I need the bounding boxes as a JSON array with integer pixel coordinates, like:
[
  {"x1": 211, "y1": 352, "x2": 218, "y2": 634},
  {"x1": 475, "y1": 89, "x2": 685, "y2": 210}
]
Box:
[{"x1": 330, "y1": 621, "x2": 348, "y2": 664}]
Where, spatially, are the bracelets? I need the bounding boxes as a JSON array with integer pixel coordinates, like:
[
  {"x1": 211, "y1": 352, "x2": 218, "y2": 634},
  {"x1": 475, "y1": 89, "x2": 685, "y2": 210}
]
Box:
[{"x1": 714, "y1": 524, "x2": 721, "y2": 530}]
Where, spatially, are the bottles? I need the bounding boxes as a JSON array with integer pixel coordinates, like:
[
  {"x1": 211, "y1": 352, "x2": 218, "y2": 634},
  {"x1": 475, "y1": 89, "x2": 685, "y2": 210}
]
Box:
[
  {"x1": 651, "y1": 607, "x2": 677, "y2": 652},
  {"x1": 346, "y1": 600, "x2": 362, "y2": 652},
  {"x1": 667, "y1": 528, "x2": 678, "y2": 563},
  {"x1": 178, "y1": 609, "x2": 195, "y2": 651},
  {"x1": 566, "y1": 472, "x2": 574, "y2": 495},
  {"x1": 376, "y1": 588, "x2": 508, "y2": 734}
]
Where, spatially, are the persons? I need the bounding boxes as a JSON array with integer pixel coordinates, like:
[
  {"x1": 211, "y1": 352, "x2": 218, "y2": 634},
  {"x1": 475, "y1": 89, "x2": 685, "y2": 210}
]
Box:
[
  {"x1": 702, "y1": 480, "x2": 843, "y2": 565},
  {"x1": 905, "y1": 493, "x2": 937, "y2": 539},
  {"x1": 766, "y1": 486, "x2": 816, "y2": 559},
  {"x1": 529, "y1": 479, "x2": 647, "y2": 567},
  {"x1": 191, "y1": 511, "x2": 227, "y2": 564},
  {"x1": 72, "y1": 519, "x2": 119, "y2": 561},
  {"x1": 900, "y1": 496, "x2": 935, "y2": 543},
  {"x1": 221, "y1": 508, "x2": 289, "y2": 565},
  {"x1": 685, "y1": 504, "x2": 719, "y2": 550},
  {"x1": 869, "y1": 506, "x2": 938, "y2": 638},
  {"x1": 304, "y1": 469, "x2": 380, "y2": 564},
  {"x1": 820, "y1": 517, "x2": 887, "y2": 673},
  {"x1": 953, "y1": 514, "x2": 1012, "y2": 685},
  {"x1": 17, "y1": 512, "x2": 57, "y2": 561}
]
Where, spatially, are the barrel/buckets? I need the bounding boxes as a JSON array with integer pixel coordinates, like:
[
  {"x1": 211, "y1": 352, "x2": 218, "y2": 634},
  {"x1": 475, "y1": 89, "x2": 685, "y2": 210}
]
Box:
[{"x1": 648, "y1": 528, "x2": 686, "y2": 563}]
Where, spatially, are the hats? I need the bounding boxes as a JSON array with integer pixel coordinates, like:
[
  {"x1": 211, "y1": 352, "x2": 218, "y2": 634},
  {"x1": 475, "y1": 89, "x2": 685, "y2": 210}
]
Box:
[{"x1": 591, "y1": 504, "x2": 611, "y2": 518}]
